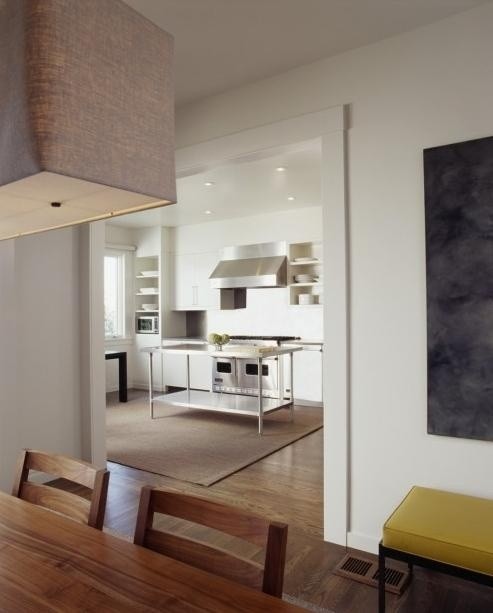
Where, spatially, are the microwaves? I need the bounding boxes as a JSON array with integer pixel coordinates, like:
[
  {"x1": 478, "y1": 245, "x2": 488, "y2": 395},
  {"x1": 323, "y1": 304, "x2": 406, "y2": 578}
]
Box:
[{"x1": 136, "y1": 316, "x2": 159, "y2": 334}]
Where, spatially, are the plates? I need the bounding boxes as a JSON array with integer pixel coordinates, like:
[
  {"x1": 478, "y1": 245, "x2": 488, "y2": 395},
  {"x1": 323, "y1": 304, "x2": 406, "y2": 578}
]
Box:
[
  {"x1": 141, "y1": 271, "x2": 158, "y2": 276},
  {"x1": 293, "y1": 274, "x2": 315, "y2": 283},
  {"x1": 140, "y1": 287, "x2": 158, "y2": 294},
  {"x1": 292, "y1": 257, "x2": 319, "y2": 262},
  {"x1": 141, "y1": 304, "x2": 157, "y2": 310}
]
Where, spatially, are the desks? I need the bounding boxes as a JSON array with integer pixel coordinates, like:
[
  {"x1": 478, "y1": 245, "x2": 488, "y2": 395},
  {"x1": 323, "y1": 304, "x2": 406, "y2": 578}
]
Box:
[
  {"x1": 0, "y1": 489, "x2": 312, "y2": 612},
  {"x1": 104, "y1": 350, "x2": 127, "y2": 402}
]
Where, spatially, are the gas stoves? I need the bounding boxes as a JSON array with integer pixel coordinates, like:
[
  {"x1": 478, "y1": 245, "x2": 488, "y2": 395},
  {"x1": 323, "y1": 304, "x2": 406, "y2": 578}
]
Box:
[{"x1": 228, "y1": 335, "x2": 301, "y2": 340}]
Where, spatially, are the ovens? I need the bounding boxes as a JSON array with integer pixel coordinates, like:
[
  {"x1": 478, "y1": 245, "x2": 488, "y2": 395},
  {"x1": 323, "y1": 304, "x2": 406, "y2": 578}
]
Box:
[{"x1": 211, "y1": 357, "x2": 280, "y2": 399}]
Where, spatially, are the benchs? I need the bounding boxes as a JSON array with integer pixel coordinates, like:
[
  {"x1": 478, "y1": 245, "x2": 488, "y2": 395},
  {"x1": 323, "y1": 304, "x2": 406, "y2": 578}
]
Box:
[{"x1": 378, "y1": 484, "x2": 492, "y2": 612}]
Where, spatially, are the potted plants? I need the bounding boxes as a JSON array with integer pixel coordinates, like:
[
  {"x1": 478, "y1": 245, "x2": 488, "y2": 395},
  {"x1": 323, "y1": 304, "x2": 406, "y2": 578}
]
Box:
[{"x1": 206, "y1": 333, "x2": 229, "y2": 351}]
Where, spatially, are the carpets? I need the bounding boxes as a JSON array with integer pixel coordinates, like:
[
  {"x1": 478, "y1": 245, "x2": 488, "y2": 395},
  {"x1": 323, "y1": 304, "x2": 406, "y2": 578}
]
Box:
[{"x1": 105, "y1": 393, "x2": 322, "y2": 488}]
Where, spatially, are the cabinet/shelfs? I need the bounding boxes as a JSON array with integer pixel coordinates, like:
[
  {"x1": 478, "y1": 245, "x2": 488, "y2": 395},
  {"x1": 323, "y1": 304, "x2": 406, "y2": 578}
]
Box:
[
  {"x1": 161, "y1": 340, "x2": 322, "y2": 402},
  {"x1": 288, "y1": 240, "x2": 322, "y2": 305},
  {"x1": 134, "y1": 254, "x2": 159, "y2": 334}
]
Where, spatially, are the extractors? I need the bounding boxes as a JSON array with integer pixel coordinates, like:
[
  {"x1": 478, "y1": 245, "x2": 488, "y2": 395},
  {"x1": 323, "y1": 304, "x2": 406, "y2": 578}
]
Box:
[{"x1": 204, "y1": 242, "x2": 290, "y2": 290}]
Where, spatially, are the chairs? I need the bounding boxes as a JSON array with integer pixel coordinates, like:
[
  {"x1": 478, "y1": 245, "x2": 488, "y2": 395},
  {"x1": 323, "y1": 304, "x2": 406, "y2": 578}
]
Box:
[
  {"x1": 135, "y1": 484, "x2": 287, "y2": 603},
  {"x1": 11, "y1": 450, "x2": 108, "y2": 532}
]
